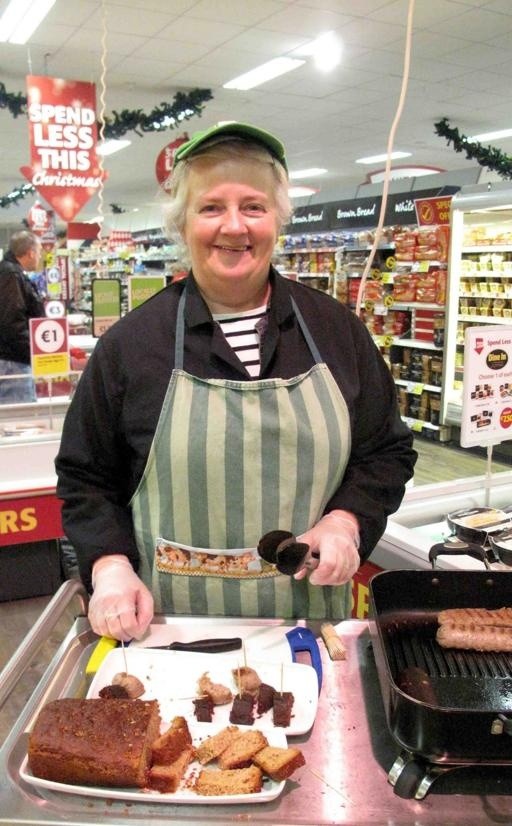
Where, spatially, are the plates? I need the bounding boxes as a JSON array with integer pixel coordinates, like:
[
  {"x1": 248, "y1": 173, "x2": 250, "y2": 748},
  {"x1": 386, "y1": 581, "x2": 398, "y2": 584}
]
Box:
[
  {"x1": 86, "y1": 645, "x2": 321, "y2": 739},
  {"x1": 20, "y1": 698, "x2": 291, "y2": 806}
]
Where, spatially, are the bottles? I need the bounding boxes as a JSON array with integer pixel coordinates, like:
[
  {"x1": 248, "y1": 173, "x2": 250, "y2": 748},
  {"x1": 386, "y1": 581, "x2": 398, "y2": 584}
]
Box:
[{"x1": 108, "y1": 229, "x2": 133, "y2": 249}]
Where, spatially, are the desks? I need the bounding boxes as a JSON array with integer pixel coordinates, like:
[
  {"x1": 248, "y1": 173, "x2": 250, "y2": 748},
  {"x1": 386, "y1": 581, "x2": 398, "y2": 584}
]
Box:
[{"x1": 0, "y1": 572, "x2": 512, "y2": 825}]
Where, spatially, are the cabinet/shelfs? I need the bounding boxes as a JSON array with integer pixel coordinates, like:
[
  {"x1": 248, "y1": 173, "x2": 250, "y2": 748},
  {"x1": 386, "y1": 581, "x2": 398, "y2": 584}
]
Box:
[
  {"x1": 0, "y1": 366, "x2": 87, "y2": 595},
  {"x1": 68, "y1": 228, "x2": 186, "y2": 338},
  {"x1": 337, "y1": 183, "x2": 512, "y2": 462},
  {"x1": 276, "y1": 201, "x2": 337, "y2": 297}
]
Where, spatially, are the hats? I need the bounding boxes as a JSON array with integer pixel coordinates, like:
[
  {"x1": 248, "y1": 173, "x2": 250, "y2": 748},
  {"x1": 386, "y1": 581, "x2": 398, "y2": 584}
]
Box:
[{"x1": 172, "y1": 118, "x2": 290, "y2": 182}]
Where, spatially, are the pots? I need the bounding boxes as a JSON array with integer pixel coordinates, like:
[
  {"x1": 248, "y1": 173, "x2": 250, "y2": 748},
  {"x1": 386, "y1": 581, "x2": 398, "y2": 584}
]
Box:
[{"x1": 369, "y1": 538, "x2": 510, "y2": 772}]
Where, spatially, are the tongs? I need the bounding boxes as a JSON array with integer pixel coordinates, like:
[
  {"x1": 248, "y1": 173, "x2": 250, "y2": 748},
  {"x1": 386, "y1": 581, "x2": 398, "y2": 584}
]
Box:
[{"x1": 256, "y1": 524, "x2": 322, "y2": 577}]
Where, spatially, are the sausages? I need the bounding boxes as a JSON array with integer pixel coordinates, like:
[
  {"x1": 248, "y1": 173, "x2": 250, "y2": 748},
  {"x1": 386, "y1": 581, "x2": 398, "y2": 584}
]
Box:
[
  {"x1": 434, "y1": 622, "x2": 512, "y2": 653},
  {"x1": 437, "y1": 607, "x2": 512, "y2": 628}
]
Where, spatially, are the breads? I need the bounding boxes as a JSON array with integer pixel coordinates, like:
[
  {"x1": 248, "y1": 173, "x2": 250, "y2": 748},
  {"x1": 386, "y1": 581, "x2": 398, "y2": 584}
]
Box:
[{"x1": 28, "y1": 666, "x2": 306, "y2": 796}]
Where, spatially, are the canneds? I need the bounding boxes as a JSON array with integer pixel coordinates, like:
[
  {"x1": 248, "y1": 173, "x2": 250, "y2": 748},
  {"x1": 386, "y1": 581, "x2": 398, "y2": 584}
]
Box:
[{"x1": 433, "y1": 313, "x2": 445, "y2": 347}]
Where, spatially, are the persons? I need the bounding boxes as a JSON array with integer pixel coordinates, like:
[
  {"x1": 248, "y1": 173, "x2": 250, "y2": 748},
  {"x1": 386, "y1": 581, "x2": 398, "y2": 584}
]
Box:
[
  {"x1": 0, "y1": 230, "x2": 47, "y2": 407},
  {"x1": 55, "y1": 122, "x2": 419, "y2": 641}
]
